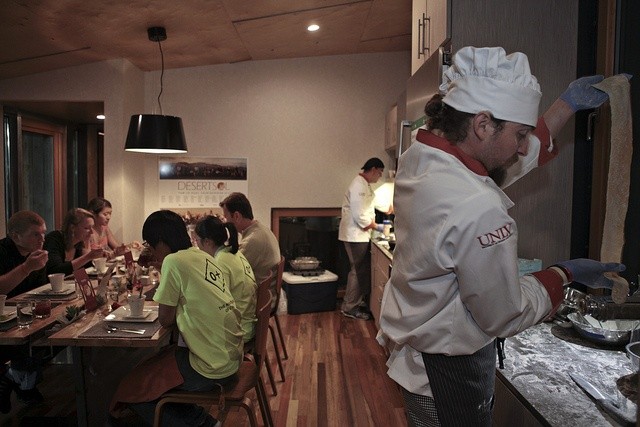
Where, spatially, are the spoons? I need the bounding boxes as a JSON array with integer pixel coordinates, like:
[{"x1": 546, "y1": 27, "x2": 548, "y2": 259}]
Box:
[{"x1": 102, "y1": 324, "x2": 145, "y2": 334}]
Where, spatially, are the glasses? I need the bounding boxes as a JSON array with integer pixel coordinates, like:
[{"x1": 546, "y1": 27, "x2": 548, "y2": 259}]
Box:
[{"x1": 142, "y1": 241, "x2": 148, "y2": 248}]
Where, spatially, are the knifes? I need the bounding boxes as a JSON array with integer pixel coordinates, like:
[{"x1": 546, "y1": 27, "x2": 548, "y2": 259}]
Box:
[{"x1": 568, "y1": 370, "x2": 638, "y2": 426}]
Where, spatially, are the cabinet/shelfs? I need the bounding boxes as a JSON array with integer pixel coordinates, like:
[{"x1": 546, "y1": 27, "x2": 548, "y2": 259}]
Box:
[{"x1": 411, "y1": 0, "x2": 455, "y2": 78}]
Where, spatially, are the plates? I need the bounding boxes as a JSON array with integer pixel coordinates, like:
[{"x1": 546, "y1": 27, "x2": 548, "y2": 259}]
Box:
[
  {"x1": 1, "y1": 306, "x2": 17, "y2": 324},
  {"x1": 24, "y1": 280, "x2": 75, "y2": 295},
  {"x1": 566, "y1": 311, "x2": 640, "y2": 343},
  {"x1": 102, "y1": 304, "x2": 159, "y2": 322},
  {"x1": 85, "y1": 266, "x2": 116, "y2": 275},
  {"x1": 122, "y1": 308, "x2": 152, "y2": 319}
]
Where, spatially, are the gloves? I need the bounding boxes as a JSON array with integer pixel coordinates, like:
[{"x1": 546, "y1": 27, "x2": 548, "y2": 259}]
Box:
[
  {"x1": 559, "y1": 73, "x2": 633, "y2": 113},
  {"x1": 557, "y1": 258, "x2": 626, "y2": 289}
]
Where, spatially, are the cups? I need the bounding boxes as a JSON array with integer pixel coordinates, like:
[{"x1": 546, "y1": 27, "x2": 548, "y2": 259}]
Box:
[
  {"x1": 34, "y1": 295, "x2": 51, "y2": 319},
  {"x1": 127, "y1": 294, "x2": 147, "y2": 316},
  {"x1": 48, "y1": 273, "x2": 65, "y2": 292},
  {"x1": 92, "y1": 257, "x2": 107, "y2": 271},
  {"x1": 16, "y1": 297, "x2": 33, "y2": 328}
]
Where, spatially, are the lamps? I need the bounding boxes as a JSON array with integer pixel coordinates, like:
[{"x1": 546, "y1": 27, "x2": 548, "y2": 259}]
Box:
[{"x1": 123, "y1": 25, "x2": 189, "y2": 157}]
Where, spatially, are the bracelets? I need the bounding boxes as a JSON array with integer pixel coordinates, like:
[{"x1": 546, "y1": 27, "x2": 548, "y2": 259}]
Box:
[{"x1": 20, "y1": 264, "x2": 30, "y2": 275}]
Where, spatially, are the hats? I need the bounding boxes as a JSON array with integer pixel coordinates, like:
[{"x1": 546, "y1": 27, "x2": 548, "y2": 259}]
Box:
[
  {"x1": 361, "y1": 158, "x2": 384, "y2": 169},
  {"x1": 438, "y1": 46, "x2": 543, "y2": 127}
]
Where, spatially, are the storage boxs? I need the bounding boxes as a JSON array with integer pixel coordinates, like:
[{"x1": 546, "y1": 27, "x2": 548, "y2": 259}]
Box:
[{"x1": 280, "y1": 269, "x2": 340, "y2": 316}]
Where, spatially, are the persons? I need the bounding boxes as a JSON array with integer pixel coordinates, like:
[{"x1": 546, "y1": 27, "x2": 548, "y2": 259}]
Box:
[
  {"x1": 376, "y1": 46, "x2": 633, "y2": 426},
  {"x1": 1, "y1": 209, "x2": 66, "y2": 413},
  {"x1": 83, "y1": 198, "x2": 143, "y2": 260},
  {"x1": 194, "y1": 215, "x2": 259, "y2": 355},
  {"x1": 337, "y1": 157, "x2": 386, "y2": 321},
  {"x1": 107, "y1": 209, "x2": 245, "y2": 426},
  {"x1": 44, "y1": 207, "x2": 111, "y2": 282},
  {"x1": 219, "y1": 191, "x2": 281, "y2": 312}
]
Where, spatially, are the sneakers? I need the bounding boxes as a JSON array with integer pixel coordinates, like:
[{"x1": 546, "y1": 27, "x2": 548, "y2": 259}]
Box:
[{"x1": 343, "y1": 310, "x2": 370, "y2": 320}]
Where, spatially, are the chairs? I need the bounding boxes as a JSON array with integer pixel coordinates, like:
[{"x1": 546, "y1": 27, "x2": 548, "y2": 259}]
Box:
[
  {"x1": 151, "y1": 289, "x2": 273, "y2": 427},
  {"x1": 242, "y1": 270, "x2": 275, "y2": 426},
  {"x1": 268, "y1": 254, "x2": 290, "y2": 383}
]
